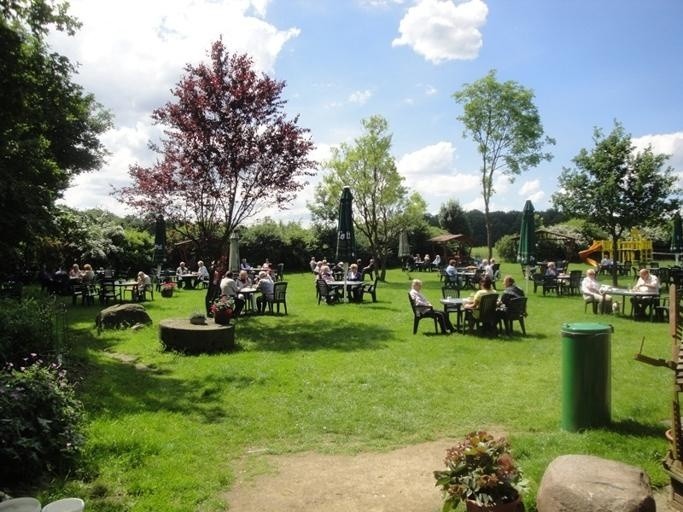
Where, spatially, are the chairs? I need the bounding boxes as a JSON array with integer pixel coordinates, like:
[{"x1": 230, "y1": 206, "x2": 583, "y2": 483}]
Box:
[
  {"x1": 43, "y1": 263, "x2": 209, "y2": 306},
  {"x1": 400, "y1": 250, "x2": 528, "y2": 338},
  {"x1": 309, "y1": 256, "x2": 378, "y2": 305},
  {"x1": 213, "y1": 263, "x2": 287, "y2": 316},
  {"x1": 532, "y1": 258, "x2": 683, "y2": 324}
]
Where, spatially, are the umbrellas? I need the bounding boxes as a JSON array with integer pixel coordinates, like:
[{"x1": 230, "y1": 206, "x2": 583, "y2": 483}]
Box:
[
  {"x1": 151, "y1": 212, "x2": 168, "y2": 270},
  {"x1": 229, "y1": 232, "x2": 240, "y2": 275},
  {"x1": 516, "y1": 200, "x2": 538, "y2": 299},
  {"x1": 334, "y1": 185, "x2": 357, "y2": 304}
]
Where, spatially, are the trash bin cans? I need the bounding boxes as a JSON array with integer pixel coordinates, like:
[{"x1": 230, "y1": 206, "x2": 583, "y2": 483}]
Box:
[{"x1": 560, "y1": 322, "x2": 614, "y2": 433}]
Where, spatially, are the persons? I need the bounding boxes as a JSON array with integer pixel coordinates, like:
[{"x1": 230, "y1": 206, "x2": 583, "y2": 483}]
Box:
[
  {"x1": 599, "y1": 253, "x2": 613, "y2": 276},
  {"x1": 399, "y1": 250, "x2": 496, "y2": 289},
  {"x1": 495, "y1": 277, "x2": 525, "y2": 339},
  {"x1": 544, "y1": 261, "x2": 560, "y2": 277},
  {"x1": 132, "y1": 270, "x2": 152, "y2": 303},
  {"x1": 176, "y1": 254, "x2": 276, "y2": 317},
  {"x1": 629, "y1": 268, "x2": 660, "y2": 322},
  {"x1": 464, "y1": 278, "x2": 498, "y2": 335},
  {"x1": 409, "y1": 279, "x2": 458, "y2": 335},
  {"x1": 38, "y1": 262, "x2": 113, "y2": 298},
  {"x1": 580, "y1": 267, "x2": 613, "y2": 314},
  {"x1": 310, "y1": 255, "x2": 375, "y2": 305}
]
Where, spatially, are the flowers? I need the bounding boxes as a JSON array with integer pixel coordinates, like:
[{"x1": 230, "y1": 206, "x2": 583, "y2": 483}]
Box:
[{"x1": 431, "y1": 429, "x2": 521, "y2": 511}]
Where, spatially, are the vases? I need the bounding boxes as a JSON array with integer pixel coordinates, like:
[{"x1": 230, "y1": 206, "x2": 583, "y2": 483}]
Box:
[{"x1": 463, "y1": 488, "x2": 524, "y2": 512}]
